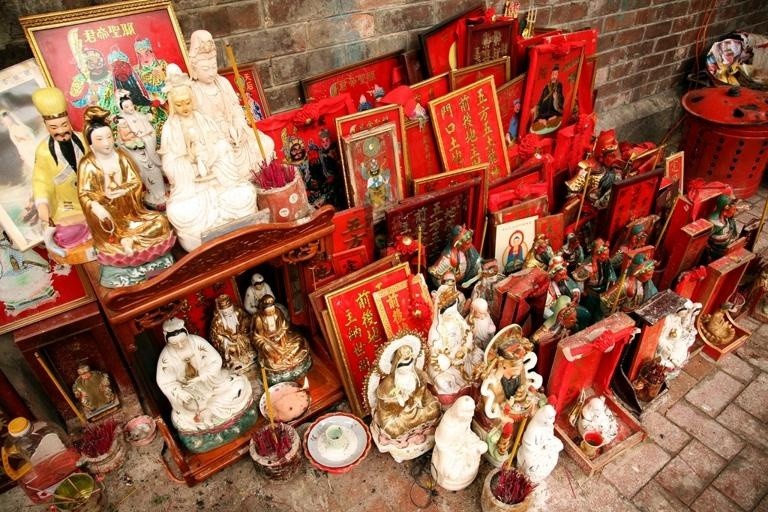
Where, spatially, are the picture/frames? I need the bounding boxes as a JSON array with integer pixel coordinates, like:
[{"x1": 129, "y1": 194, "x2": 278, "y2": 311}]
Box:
[
  {"x1": 1, "y1": 59, "x2": 57, "y2": 253},
  {"x1": 218, "y1": 62, "x2": 269, "y2": 125},
  {"x1": 343, "y1": 121, "x2": 403, "y2": 222},
  {"x1": 19, "y1": 0, "x2": 190, "y2": 146},
  {"x1": 300, "y1": 48, "x2": 415, "y2": 105}
]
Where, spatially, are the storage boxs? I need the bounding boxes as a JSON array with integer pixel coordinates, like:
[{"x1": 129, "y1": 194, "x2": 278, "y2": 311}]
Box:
[
  {"x1": 547, "y1": 311, "x2": 646, "y2": 476},
  {"x1": 690, "y1": 246, "x2": 754, "y2": 361},
  {"x1": 612, "y1": 287, "x2": 687, "y2": 417}
]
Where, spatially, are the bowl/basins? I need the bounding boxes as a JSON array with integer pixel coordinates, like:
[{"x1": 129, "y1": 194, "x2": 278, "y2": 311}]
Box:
[
  {"x1": 258, "y1": 381, "x2": 311, "y2": 425},
  {"x1": 303, "y1": 412, "x2": 371, "y2": 474},
  {"x1": 308, "y1": 416, "x2": 367, "y2": 468},
  {"x1": 123, "y1": 415, "x2": 156, "y2": 447}
]
